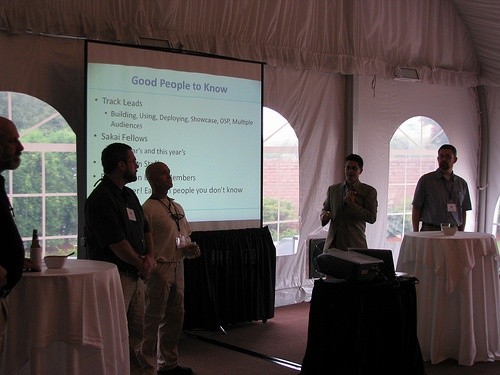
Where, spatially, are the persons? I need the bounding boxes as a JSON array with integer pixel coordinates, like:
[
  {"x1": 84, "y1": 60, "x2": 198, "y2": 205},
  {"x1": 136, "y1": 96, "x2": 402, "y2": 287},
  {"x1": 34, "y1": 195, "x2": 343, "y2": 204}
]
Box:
[
  {"x1": 83, "y1": 143, "x2": 154, "y2": 375},
  {"x1": 0, "y1": 117, "x2": 23, "y2": 366},
  {"x1": 319, "y1": 153, "x2": 376, "y2": 253},
  {"x1": 412, "y1": 145, "x2": 473, "y2": 234},
  {"x1": 142, "y1": 161, "x2": 201, "y2": 375}
]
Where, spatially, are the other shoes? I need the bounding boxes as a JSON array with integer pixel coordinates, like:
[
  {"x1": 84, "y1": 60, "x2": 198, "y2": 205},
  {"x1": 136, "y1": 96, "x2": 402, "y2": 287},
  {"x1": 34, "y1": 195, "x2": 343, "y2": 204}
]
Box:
[{"x1": 157, "y1": 365, "x2": 193, "y2": 375}]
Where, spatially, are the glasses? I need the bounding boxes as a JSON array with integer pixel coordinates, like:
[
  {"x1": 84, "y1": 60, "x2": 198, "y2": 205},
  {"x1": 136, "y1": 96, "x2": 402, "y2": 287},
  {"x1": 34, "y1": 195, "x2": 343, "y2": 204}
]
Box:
[
  {"x1": 344, "y1": 166, "x2": 361, "y2": 170},
  {"x1": 125, "y1": 161, "x2": 139, "y2": 166}
]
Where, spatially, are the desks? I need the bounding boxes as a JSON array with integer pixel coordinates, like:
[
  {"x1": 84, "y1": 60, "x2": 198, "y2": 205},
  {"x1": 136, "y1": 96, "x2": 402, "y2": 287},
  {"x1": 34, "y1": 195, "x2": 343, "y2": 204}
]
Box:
[
  {"x1": 0, "y1": 258, "x2": 133, "y2": 375},
  {"x1": 301, "y1": 276, "x2": 425, "y2": 375},
  {"x1": 181, "y1": 226, "x2": 277, "y2": 326},
  {"x1": 396, "y1": 231, "x2": 500, "y2": 365}
]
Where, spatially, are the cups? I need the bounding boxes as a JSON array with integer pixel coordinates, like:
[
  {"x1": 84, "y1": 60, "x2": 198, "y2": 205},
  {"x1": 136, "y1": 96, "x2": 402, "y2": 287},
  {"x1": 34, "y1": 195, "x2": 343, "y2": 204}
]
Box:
[
  {"x1": 443, "y1": 227, "x2": 457, "y2": 235},
  {"x1": 441, "y1": 223, "x2": 450, "y2": 235},
  {"x1": 184, "y1": 242, "x2": 196, "y2": 259}
]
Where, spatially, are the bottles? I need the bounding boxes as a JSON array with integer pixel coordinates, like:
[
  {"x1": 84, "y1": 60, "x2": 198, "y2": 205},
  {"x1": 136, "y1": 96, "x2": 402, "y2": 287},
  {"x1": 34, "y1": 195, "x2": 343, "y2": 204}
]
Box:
[{"x1": 29, "y1": 229, "x2": 43, "y2": 272}]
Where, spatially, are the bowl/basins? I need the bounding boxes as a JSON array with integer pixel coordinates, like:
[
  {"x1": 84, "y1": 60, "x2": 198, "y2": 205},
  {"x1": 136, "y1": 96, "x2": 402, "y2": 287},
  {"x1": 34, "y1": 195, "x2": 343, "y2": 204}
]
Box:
[{"x1": 44, "y1": 255, "x2": 68, "y2": 270}]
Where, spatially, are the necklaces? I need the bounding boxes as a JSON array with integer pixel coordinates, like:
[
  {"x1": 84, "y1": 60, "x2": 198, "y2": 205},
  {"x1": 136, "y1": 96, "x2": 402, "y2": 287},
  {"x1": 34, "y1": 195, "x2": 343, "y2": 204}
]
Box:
[{"x1": 160, "y1": 200, "x2": 181, "y2": 232}]
